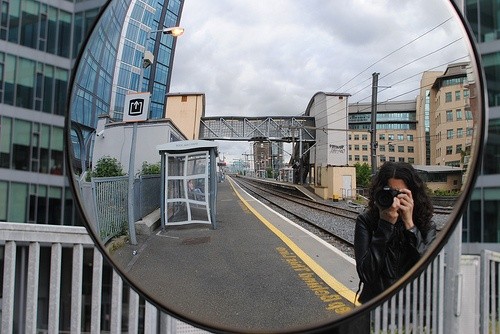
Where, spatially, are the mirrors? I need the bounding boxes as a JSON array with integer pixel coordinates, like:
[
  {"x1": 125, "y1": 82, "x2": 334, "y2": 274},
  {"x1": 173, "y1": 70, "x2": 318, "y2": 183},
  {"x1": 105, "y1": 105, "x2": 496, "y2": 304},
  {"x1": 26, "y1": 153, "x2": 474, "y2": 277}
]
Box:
[{"x1": 61, "y1": 0, "x2": 490, "y2": 334}]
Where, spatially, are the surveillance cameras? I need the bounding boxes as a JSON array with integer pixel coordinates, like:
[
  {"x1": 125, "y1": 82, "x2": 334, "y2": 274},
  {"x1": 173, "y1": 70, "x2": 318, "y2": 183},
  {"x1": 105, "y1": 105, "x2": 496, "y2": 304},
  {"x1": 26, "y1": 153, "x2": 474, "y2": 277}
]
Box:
[{"x1": 144, "y1": 51, "x2": 155, "y2": 68}]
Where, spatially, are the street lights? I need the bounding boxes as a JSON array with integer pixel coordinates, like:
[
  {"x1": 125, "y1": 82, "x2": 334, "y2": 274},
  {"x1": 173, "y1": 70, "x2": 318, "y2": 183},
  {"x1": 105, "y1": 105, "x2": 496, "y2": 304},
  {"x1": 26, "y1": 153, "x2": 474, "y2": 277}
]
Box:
[{"x1": 127, "y1": 25, "x2": 185, "y2": 246}]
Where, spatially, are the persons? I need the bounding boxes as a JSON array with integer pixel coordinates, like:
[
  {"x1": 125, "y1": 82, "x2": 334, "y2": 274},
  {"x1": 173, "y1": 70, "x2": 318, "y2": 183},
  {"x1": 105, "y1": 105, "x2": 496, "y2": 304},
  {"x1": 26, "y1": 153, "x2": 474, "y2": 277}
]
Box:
[
  {"x1": 353, "y1": 160, "x2": 438, "y2": 306},
  {"x1": 189, "y1": 179, "x2": 205, "y2": 200}
]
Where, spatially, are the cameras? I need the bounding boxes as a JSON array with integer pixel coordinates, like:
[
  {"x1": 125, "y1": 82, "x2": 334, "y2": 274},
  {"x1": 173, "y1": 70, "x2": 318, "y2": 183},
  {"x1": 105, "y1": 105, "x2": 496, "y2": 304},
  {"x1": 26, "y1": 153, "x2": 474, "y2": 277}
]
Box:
[{"x1": 374, "y1": 185, "x2": 406, "y2": 209}]
